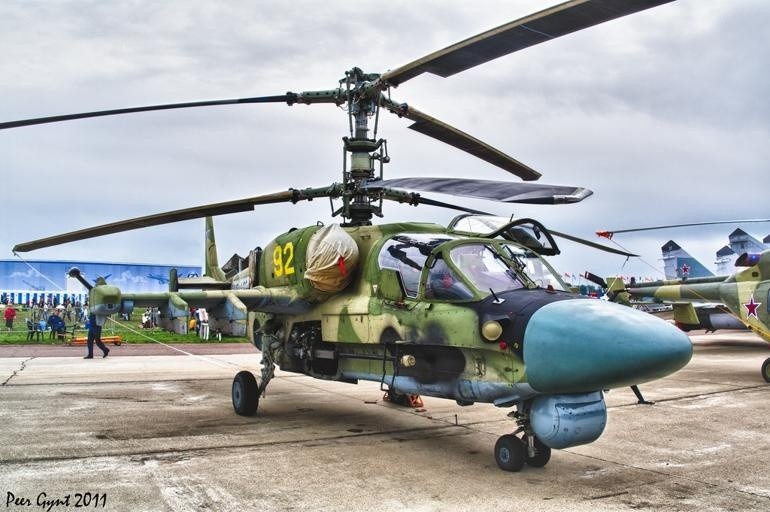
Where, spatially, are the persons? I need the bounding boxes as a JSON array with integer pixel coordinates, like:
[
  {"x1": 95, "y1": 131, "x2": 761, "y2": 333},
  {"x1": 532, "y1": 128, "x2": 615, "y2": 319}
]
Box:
[
  {"x1": 4, "y1": 303, "x2": 16, "y2": 333},
  {"x1": 24, "y1": 296, "x2": 89, "y2": 341},
  {"x1": 188, "y1": 307, "x2": 210, "y2": 340},
  {"x1": 84, "y1": 314, "x2": 110, "y2": 359}
]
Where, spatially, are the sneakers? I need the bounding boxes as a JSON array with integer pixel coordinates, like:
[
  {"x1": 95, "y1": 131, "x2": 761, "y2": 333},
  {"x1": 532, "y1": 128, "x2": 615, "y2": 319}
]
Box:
[
  {"x1": 85, "y1": 355, "x2": 93, "y2": 358},
  {"x1": 103, "y1": 349, "x2": 110, "y2": 358}
]
